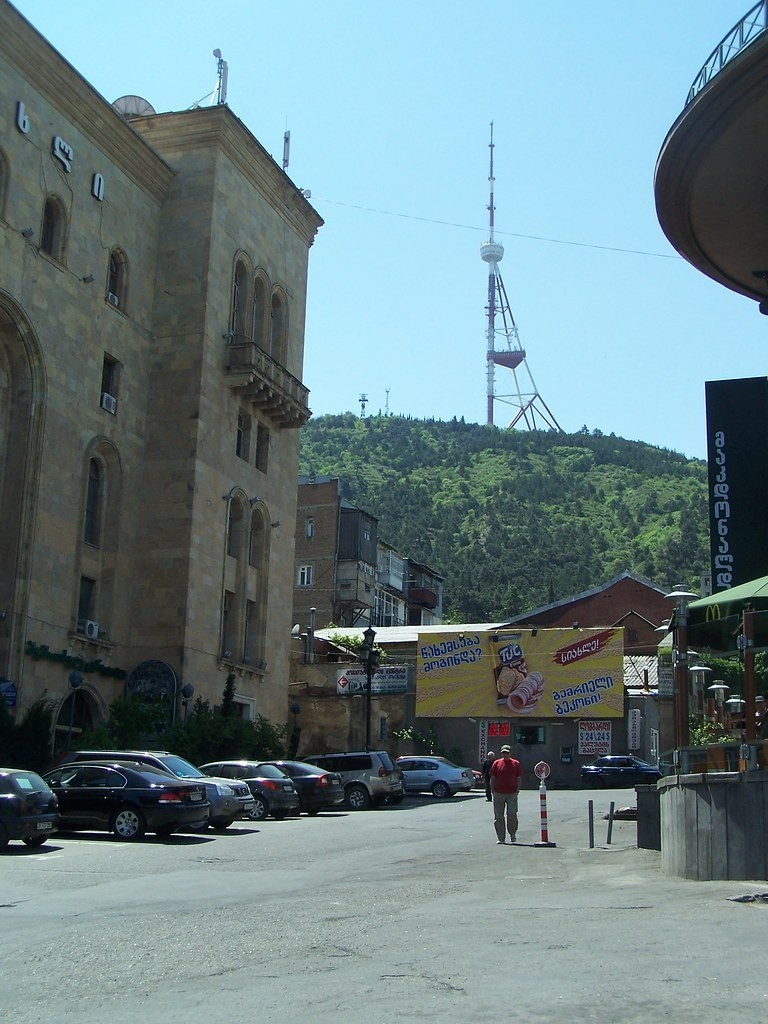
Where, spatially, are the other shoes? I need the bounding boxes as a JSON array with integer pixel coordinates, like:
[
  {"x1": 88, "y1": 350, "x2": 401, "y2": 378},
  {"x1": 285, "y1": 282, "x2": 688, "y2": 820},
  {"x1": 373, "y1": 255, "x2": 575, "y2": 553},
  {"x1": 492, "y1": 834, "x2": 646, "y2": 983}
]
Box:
[
  {"x1": 511, "y1": 834, "x2": 516, "y2": 842},
  {"x1": 499, "y1": 839, "x2": 505, "y2": 844}
]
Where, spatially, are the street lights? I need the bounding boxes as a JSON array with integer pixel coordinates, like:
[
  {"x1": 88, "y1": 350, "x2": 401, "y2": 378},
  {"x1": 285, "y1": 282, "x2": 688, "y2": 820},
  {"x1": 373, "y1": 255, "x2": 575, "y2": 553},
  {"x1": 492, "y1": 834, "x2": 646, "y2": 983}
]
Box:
[{"x1": 364, "y1": 623, "x2": 377, "y2": 756}]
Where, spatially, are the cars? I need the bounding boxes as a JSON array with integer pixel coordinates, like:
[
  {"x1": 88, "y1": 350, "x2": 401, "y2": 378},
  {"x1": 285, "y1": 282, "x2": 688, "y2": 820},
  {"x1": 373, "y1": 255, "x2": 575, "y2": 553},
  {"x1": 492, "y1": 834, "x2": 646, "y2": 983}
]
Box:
[
  {"x1": 580, "y1": 754, "x2": 662, "y2": 790},
  {"x1": 196, "y1": 759, "x2": 299, "y2": 820},
  {"x1": 0, "y1": 767, "x2": 61, "y2": 847},
  {"x1": 262, "y1": 761, "x2": 344, "y2": 815},
  {"x1": 395, "y1": 758, "x2": 474, "y2": 799},
  {"x1": 41, "y1": 760, "x2": 211, "y2": 841}
]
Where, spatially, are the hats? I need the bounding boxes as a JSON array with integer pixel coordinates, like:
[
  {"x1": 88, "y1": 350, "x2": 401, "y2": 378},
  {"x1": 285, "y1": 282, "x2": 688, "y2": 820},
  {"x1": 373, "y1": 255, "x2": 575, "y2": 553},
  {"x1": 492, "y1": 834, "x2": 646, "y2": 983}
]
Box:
[{"x1": 500, "y1": 744, "x2": 511, "y2": 753}]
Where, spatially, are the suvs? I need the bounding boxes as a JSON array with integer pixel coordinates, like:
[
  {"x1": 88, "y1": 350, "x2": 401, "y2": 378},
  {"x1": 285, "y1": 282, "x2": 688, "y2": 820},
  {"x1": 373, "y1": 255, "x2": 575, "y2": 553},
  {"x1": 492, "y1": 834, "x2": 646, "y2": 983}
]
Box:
[
  {"x1": 396, "y1": 755, "x2": 482, "y2": 789},
  {"x1": 59, "y1": 748, "x2": 255, "y2": 832},
  {"x1": 301, "y1": 750, "x2": 405, "y2": 809}
]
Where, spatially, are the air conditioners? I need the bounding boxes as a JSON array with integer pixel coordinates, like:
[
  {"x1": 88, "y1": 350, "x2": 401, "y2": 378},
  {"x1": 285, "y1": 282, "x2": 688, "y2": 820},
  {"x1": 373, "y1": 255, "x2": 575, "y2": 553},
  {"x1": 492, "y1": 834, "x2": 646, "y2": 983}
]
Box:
[
  {"x1": 109, "y1": 290, "x2": 119, "y2": 307},
  {"x1": 79, "y1": 619, "x2": 99, "y2": 640},
  {"x1": 101, "y1": 393, "x2": 117, "y2": 414}
]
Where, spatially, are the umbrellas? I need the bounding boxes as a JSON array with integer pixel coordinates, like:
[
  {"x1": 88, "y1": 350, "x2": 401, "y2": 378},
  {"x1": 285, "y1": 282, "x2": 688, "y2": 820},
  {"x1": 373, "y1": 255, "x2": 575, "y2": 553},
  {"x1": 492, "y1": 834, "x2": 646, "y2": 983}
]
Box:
[{"x1": 669, "y1": 574, "x2": 767, "y2": 660}]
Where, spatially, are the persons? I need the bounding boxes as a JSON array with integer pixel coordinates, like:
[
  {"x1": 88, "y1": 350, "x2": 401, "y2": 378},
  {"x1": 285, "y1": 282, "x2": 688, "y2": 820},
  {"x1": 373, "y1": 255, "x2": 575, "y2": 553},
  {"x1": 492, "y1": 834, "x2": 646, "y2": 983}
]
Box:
[
  {"x1": 482, "y1": 751, "x2": 496, "y2": 802},
  {"x1": 489, "y1": 745, "x2": 523, "y2": 845}
]
